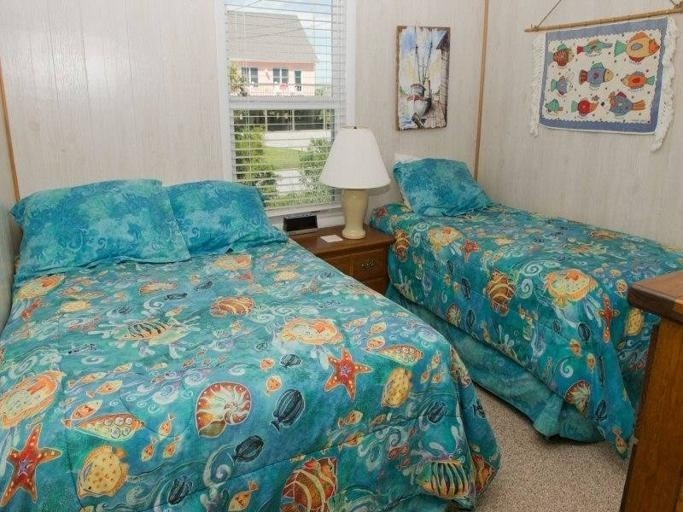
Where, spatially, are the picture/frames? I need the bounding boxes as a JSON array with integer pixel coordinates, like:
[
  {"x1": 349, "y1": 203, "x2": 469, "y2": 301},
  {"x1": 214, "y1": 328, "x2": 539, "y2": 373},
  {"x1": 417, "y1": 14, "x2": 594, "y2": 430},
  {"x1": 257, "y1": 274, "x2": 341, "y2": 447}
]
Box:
[{"x1": 396, "y1": 26, "x2": 450, "y2": 130}]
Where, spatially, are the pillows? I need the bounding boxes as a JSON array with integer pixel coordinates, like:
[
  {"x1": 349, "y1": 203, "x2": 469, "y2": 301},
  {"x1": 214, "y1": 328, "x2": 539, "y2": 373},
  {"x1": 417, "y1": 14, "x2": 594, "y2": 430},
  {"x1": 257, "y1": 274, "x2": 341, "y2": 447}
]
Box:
[
  {"x1": 8, "y1": 178, "x2": 191, "y2": 288},
  {"x1": 395, "y1": 153, "x2": 421, "y2": 209},
  {"x1": 393, "y1": 157, "x2": 493, "y2": 217},
  {"x1": 163, "y1": 180, "x2": 289, "y2": 258}
]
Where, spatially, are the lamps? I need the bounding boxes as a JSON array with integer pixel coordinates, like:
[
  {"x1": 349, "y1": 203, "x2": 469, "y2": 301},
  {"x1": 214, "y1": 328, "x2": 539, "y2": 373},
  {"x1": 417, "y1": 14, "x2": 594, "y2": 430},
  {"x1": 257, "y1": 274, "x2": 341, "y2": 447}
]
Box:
[{"x1": 318, "y1": 126, "x2": 391, "y2": 240}]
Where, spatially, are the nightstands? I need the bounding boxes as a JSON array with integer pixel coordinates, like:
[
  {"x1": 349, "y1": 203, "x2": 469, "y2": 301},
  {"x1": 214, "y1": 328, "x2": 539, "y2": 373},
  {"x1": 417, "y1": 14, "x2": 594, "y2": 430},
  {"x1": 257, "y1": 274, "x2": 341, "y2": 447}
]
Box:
[{"x1": 288, "y1": 224, "x2": 396, "y2": 297}]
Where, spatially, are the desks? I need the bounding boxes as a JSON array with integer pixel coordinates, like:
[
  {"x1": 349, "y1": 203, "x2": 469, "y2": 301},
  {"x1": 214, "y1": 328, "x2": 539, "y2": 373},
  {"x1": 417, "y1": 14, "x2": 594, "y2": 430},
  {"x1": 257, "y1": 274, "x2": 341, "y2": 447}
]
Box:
[{"x1": 619, "y1": 271, "x2": 683, "y2": 512}]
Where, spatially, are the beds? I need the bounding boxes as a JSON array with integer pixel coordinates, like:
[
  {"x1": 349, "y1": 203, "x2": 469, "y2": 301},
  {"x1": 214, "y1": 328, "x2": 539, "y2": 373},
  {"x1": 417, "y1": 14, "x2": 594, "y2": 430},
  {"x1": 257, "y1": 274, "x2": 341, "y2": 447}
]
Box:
[
  {"x1": 367, "y1": 153, "x2": 683, "y2": 460},
  {"x1": 0, "y1": 178, "x2": 500, "y2": 512}
]
